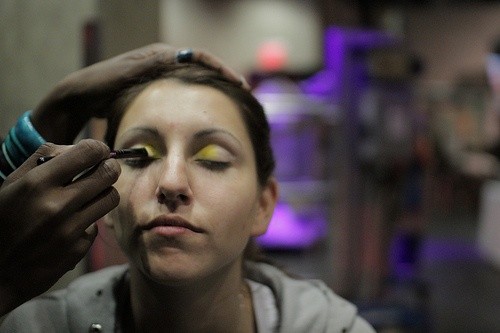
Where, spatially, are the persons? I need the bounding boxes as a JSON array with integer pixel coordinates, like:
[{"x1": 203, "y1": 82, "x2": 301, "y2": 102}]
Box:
[
  {"x1": 1, "y1": 43, "x2": 248, "y2": 333},
  {"x1": 1, "y1": 62, "x2": 379, "y2": 333}
]
